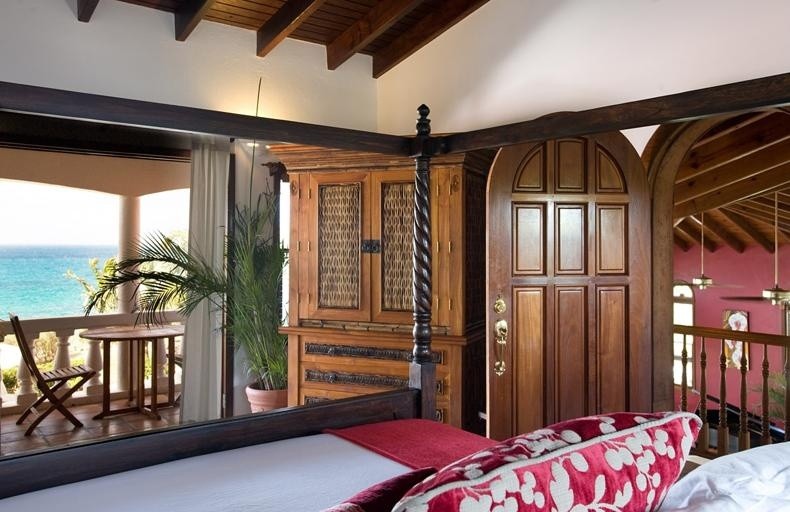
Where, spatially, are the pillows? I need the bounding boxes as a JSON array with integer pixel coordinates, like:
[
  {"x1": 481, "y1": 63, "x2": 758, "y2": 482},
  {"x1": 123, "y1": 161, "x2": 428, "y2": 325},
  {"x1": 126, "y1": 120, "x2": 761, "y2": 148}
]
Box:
[{"x1": 326, "y1": 408, "x2": 790, "y2": 511}]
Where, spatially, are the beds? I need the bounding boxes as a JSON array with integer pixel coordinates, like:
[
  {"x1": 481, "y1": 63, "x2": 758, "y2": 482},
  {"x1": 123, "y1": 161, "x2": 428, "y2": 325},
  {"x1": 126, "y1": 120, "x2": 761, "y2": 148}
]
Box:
[{"x1": 2, "y1": 104, "x2": 790, "y2": 512}]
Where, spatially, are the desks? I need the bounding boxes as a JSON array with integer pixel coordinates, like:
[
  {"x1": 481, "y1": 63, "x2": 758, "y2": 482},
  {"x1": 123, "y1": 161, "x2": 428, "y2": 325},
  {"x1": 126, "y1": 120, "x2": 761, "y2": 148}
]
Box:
[{"x1": 78, "y1": 324, "x2": 184, "y2": 423}]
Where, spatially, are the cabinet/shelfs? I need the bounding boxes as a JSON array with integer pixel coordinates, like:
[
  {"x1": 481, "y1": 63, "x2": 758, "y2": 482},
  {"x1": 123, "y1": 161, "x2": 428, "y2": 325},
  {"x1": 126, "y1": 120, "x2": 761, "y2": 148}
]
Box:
[{"x1": 283, "y1": 166, "x2": 484, "y2": 435}]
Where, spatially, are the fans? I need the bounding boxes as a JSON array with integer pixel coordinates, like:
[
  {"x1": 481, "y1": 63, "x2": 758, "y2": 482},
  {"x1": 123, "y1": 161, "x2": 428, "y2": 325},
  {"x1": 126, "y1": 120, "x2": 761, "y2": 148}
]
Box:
[{"x1": 673, "y1": 194, "x2": 790, "y2": 309}]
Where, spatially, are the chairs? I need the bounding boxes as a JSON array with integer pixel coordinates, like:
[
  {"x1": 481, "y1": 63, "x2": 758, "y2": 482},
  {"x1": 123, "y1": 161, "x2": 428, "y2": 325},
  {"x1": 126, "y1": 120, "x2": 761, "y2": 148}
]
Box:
[{"x1": 6, "y1": 310, "x2": 97, "y2": 436}]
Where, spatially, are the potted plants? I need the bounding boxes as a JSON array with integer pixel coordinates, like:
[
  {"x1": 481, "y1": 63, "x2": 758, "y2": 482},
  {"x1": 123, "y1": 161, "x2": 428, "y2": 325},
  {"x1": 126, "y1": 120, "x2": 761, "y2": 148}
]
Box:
[{"x1": 79, "y1": 76, "x2": 289, "y2": 416}]
